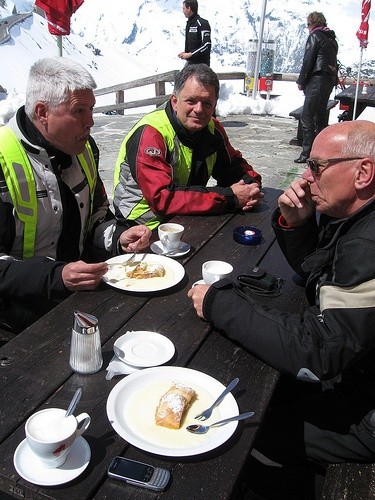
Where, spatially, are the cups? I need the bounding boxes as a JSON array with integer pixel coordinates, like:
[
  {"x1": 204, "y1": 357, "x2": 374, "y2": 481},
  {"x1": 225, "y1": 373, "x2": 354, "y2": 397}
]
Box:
[
  {"x1": 158, "y1": 222, "x2": 185, "y2": 249},
  {"x1": 202, "y1": 261, "x2": 241, "y2": 290},
  {"x1": 24, "y1": 407, "x2": 92, "y2": 461},
  {"x1": 362, "y1": 85, "x2": 369, "y2": 94}
]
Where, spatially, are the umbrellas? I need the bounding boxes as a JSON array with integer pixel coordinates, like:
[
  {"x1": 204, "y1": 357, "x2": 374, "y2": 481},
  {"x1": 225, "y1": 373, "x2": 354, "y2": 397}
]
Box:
[
  {"x1": 35, "y1": 0, "x2": 85, "y2": 58},
  {"x1": 353, "y1": 0, "x2": 371, "y2": 120}
]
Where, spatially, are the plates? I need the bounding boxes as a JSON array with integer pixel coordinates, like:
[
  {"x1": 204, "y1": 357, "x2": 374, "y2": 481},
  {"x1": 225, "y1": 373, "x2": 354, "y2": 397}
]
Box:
[
  {"x1": 105, "y1": 366, "x2": 239, "y2": 457},
  {"x1": 112, "y1": 331, "x2": 177, "y2": 368},
  {"x1": 150, "y1": 239, "x2": 191, "y2": 258},
  {"x1": 13, "y1": 436, "x2": 91, "y2": 486},
  {"x1": 101, "y1": 253, "x2": 185, "y2": 293}
]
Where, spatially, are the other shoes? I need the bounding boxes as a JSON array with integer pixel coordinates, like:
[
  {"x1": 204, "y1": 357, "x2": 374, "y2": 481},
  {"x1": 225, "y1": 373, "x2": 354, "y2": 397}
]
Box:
[{"x1": 294, "y1": 158, "x2": 306, "y2": 163}]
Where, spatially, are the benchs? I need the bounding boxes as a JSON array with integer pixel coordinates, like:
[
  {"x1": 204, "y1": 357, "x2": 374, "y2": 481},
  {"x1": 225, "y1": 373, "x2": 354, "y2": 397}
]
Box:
[{"x1": 289, "y1": 99, "x2": 339, "y2": 146}]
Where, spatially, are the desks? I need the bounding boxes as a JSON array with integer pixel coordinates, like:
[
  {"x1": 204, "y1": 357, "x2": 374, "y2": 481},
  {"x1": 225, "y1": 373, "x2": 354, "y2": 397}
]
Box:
[
  {"x1": 334, "y1": 82, "x2": 375, "y2": 123},
  {"x1": 0, "y1": 185, "x2": 327, "y2": 500}
]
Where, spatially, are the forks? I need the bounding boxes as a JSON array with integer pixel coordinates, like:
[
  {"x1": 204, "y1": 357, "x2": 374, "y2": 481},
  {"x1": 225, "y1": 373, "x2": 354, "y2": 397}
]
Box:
[
  {"x1": 107, "y1": 260, "x2": 142, "y2": 268},
  {"x1": 195, "y1": 378, "x2": 239, "y2": 423}
]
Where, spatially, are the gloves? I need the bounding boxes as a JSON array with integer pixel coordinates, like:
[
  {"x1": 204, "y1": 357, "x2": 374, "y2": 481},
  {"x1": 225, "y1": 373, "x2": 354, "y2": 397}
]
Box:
[{"x1": 298, "y1": 84, "x2": 303, "y2": 90}]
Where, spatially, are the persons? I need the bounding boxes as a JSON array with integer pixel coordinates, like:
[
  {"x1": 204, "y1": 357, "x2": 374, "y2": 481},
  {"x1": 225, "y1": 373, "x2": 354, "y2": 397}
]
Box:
[
  {"x1": 187, "y1": 121, "x2": 375, "y2": 493},
  {"x1": 113, "y1": 64, "x2": 264, "y2": 236},
  {"x1": 0, "y1": 57, "x2": 152, "y2": 347},
  {"x1": 296, "y1": 12, "x2": 339, "y2": 165},
  {"x1": 178, "y1": 0, "x2": 212, "y2": 67}
]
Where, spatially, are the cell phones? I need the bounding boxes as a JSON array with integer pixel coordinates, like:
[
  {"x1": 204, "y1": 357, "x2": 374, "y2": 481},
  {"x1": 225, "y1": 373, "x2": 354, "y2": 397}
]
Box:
[{"x1": 108, "y1": 456, "x2": 170, "y2": 491}]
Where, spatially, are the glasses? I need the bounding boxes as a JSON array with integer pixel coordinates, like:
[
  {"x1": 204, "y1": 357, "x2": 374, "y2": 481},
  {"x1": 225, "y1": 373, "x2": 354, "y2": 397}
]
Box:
[
  {"x1": 306, "y1": 156, "x2": 364, "y2": 179},
  {"x1": 307, "y1": 22, "x2": 311, "y2": 26}
]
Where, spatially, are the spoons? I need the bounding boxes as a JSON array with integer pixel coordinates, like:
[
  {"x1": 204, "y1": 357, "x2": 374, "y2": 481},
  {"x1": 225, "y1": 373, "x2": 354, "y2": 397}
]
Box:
[{"x1": 186, "y1": 410, "x2": 254, "y2": 433}]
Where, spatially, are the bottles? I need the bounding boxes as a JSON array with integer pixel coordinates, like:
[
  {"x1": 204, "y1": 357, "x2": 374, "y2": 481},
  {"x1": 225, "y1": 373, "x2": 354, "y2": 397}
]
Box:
[{"x1": 68, "y1": 310, "x2": 104, "y2": 375}]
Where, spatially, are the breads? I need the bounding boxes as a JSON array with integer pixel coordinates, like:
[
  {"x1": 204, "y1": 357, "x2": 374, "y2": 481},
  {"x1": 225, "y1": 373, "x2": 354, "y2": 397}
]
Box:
[
  {"x1": 155, "y1": 382, "x2": 196, "y2": 430},
  {"x1": 126, "y1": 262, "x2": 165, "y2": 279}
]
